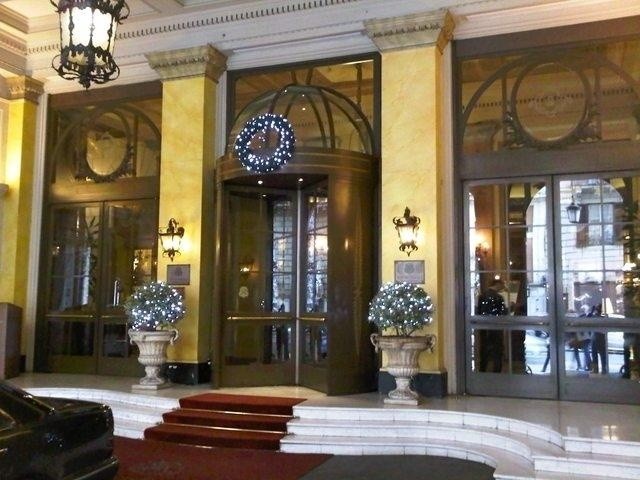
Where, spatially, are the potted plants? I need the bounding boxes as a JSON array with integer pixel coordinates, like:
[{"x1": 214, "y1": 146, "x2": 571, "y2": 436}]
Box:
[
  {"x1": 123, "y1": 282, "x2": 186, "y2": 389},
  {"x1": 369, "y1": 282, "x2": 437, "y2": 406}
]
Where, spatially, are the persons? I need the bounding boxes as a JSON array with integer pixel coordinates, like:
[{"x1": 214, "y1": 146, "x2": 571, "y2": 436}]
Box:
[
  {"x1": 477, "y1": 278, "x2": 523, "y2": 372},
  {"x1": 308, "y1": 304, "x2": 327, "y2": 362},
  {"x1": 277, "y1": 304, "x2": 290, "y2": 363},
  {"x1": 539, "y1": 304, "x2": 608, "y2": 375}
]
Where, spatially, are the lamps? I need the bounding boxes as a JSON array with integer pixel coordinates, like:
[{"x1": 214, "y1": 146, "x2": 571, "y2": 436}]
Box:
[
  {"x1": 238, "y1": 256, "x2": 254, "y2": 281},
  {"x1": 157, "y1": 218, "x2": 184, "y2": 262},
  {"x1": 394, "y1": 212, "x2": 420, "y2": 257},
  {"x1": 51, "y1": 0, "x2": 130, "y2": 89}
]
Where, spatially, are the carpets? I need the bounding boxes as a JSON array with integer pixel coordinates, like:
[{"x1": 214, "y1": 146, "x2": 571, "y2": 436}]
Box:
[{"x1": 113, "y1": 437, "x2": 334, "y2": 480}]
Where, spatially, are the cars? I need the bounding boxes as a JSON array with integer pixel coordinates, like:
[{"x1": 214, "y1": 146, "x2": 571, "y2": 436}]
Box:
[{"x1": 0, "y1": 380, "x2": 119, "y2": 480}]
